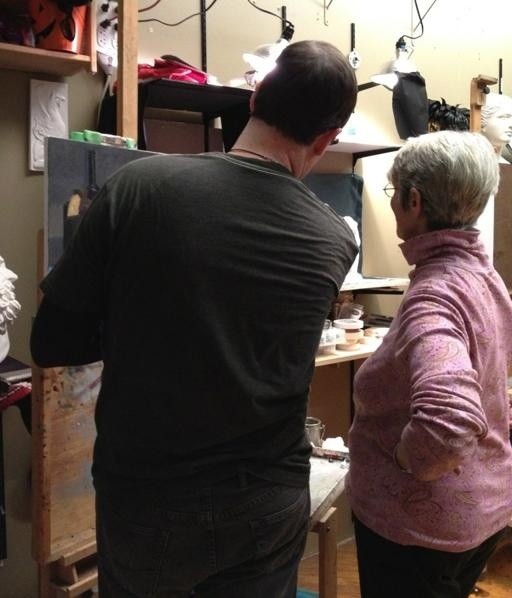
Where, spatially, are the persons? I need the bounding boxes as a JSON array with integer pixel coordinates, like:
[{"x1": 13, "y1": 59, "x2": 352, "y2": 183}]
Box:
[
  {"x1": 28, "y1": 39, "x2": 357, "y2": 598},
  {"x1": 343, "y1": 129, "x2": 511, "y2": 598},
  {"x1": 481, "y1": 93, "x2": 512, "y2": 163}
]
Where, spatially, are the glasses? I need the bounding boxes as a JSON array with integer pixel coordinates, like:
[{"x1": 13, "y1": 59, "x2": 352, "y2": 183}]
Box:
[{"x1": 381, "y1": 182, "x2": 398, "y2": 199}]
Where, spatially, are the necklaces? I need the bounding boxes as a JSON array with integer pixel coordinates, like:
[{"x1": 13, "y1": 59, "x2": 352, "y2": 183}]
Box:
[{"x1": 228, "y1": 148, "x2": 271, "y2": 161}]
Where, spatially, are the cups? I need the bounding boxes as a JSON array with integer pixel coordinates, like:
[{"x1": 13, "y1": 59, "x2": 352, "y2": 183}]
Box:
[{"x1": 305, "y1": 416, "x2": 327, "y2": 448}]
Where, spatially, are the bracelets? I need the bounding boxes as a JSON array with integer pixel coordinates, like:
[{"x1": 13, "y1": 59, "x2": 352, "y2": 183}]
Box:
[{"x1": 392, "y1": 442, "x2": 412, "y2": 474}]
